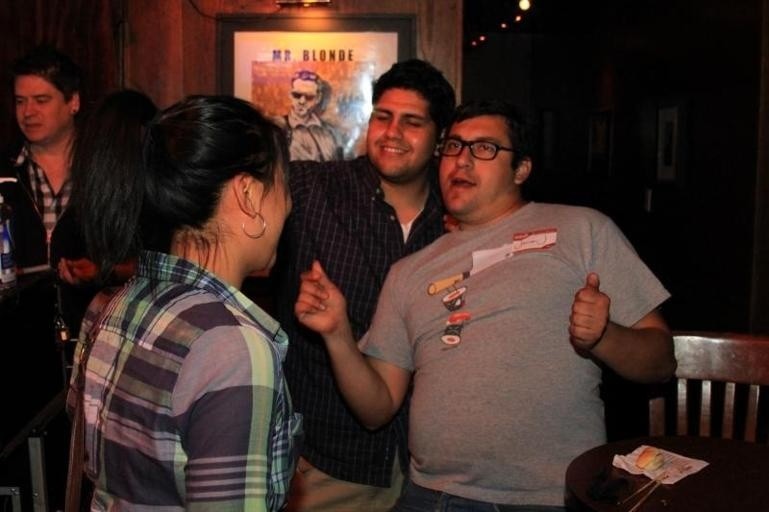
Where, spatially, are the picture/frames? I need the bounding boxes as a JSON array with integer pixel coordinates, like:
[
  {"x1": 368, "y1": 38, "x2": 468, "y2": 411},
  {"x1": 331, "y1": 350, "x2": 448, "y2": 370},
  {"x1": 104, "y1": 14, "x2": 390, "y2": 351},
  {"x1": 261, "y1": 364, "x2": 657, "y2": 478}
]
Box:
[{"x1": 214, "y1": 11, "x2": 418, "y2": 163}]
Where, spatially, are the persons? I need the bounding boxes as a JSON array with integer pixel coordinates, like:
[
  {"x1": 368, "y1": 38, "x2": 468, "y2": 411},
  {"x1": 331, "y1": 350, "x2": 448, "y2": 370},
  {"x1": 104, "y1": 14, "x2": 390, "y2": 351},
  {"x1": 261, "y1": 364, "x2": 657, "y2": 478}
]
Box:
[
  {"x1": 64, "y1": 90, "x2": 305, "y2": 512},
  {"x1": 273, "y1": 70, "x2": 345, "y2": 161},
  {"x1": 294, "y1": 98, "x2": 678, "y2": 512},
  {"x1": 0, "y1": 60, "x2": 137, "y2": 285},
  {"x1": 235, "y1": 59, "x2": 457, "y2": 512}
]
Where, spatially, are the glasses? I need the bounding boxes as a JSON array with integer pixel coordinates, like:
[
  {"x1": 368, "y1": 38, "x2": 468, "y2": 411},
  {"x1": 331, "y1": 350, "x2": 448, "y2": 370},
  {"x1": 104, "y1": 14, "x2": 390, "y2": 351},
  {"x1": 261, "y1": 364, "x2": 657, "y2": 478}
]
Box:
[
  {"x1": 437, "y1": 137, "x2": 515, "y2": 160},
  {"x1": 292, "y1": 92, "x2": 317, "y2": 101}
]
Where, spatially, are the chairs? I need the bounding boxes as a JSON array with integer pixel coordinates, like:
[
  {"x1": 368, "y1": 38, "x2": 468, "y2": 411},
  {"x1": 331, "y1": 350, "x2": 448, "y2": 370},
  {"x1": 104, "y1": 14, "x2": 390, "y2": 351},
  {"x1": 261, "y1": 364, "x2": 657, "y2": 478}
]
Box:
[{"x1": 646, "y1": 331, "x2": 769, "y2": 442}]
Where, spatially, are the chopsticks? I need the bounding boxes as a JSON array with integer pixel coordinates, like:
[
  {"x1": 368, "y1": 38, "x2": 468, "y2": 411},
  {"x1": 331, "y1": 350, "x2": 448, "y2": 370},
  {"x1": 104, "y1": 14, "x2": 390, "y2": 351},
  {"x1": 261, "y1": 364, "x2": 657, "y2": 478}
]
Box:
[{"x1": 622, "y1": 471, "x2": 670, "y2": 512}]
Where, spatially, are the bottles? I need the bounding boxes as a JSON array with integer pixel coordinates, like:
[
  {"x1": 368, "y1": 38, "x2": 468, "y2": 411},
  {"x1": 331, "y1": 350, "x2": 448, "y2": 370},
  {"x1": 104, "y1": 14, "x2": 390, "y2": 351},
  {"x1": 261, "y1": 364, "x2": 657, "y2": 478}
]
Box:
[{"x1": 0, "y1": 225, "x2": 17, "y2": 284}]
